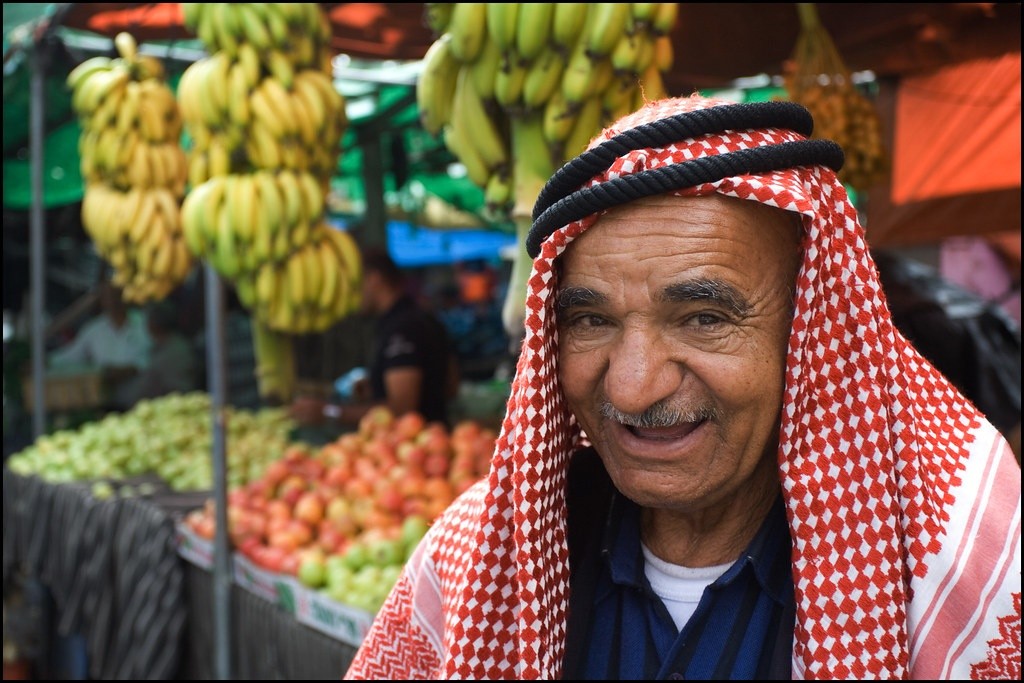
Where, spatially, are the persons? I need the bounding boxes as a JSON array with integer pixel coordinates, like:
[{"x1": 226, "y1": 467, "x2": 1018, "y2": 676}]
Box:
[
  {"x1": 38, "y1": 261, "x2": 158, "y2": 371},
  {"x1": 336, "y1": 90, "x2": 1022, "y2": 683},
  {"x1": 287, "y1": 258, "x2": 460, "y2": 433}
]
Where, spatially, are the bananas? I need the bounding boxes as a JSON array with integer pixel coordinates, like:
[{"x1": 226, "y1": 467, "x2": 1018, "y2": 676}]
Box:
[{"x1": 68, "y1": 3, "x2": 680, "y2": 338}]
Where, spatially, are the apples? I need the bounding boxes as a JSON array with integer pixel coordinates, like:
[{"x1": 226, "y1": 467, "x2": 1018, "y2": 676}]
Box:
[{"x1": 186, "y1": 407, "x2": 496, "y2": 610}]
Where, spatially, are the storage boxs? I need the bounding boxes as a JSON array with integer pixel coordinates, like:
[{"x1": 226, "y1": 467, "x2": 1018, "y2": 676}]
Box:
[{"x1": 18, "y1": 373, "x2": 109, "y2": 415}]
